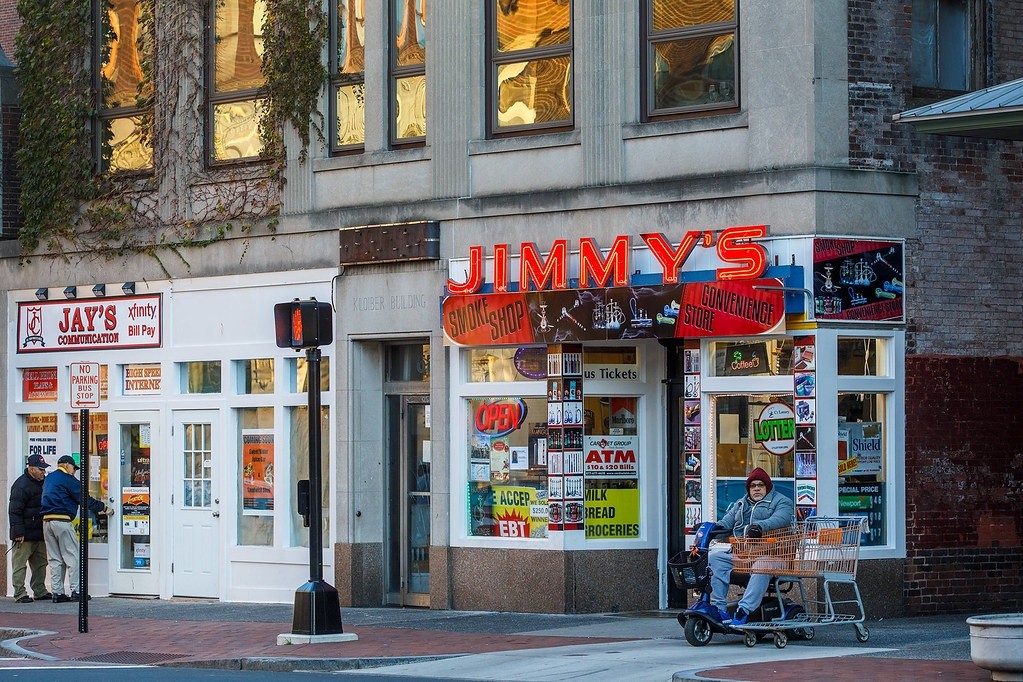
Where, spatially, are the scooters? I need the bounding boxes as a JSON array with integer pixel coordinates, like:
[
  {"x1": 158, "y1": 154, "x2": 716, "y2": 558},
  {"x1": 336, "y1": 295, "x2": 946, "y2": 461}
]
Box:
[{"x1": 667, "y1": 527, "x2": 808, "y2": 647}]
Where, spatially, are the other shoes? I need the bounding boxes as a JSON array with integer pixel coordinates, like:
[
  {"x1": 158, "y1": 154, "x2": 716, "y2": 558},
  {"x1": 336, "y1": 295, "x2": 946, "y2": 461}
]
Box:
[
  {"x1": 15, "y1": 596, "x2": 33, "y2": 603},
  {"x1": 53, "y1": 593, "x2": 72, "y2": 603},
  {"x1": 71, "y1": 591, "x2": 91, "y2": 601},
  {"x1": 34, "y1": 593, "x2": 52, "y2": 600}
]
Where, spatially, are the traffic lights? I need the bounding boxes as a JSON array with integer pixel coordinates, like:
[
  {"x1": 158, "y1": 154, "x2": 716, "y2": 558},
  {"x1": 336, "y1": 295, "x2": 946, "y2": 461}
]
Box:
[{"x1": 274, "y1": 302, "x2": 332, "y2": 349}]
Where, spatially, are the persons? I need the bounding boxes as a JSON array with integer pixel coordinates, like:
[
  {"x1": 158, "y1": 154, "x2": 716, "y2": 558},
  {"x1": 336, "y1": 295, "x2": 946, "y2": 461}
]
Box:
[
  {"x1": 41, "y1": 456, "x2": 114, "y2": 603},
  {"x1": 692, "y1": 468, "x2": 793, "y2": 625},
  {"x1": 9, "y1": 454, "x2": 53, "y2": 603}
]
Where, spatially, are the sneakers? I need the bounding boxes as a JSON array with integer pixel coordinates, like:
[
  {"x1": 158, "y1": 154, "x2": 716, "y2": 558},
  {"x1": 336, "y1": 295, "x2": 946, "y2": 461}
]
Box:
[
  {"x1": 729, "y1": 608, "x2": 747, "y2": 625},
  {"x1": 718, "y1": 608, "x2": 733, "y2": 623}
]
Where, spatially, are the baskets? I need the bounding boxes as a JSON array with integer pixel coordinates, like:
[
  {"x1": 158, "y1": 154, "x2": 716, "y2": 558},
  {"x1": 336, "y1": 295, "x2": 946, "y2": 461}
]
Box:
[{"x1": 668, "y1": 552, "x2": 707, "y2": 588}]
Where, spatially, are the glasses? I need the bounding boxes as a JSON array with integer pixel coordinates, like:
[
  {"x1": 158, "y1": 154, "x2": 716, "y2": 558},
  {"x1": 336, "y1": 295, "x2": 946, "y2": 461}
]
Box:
[{"x1": 750, "y1": 483, "x2": 765, "y2": 488}]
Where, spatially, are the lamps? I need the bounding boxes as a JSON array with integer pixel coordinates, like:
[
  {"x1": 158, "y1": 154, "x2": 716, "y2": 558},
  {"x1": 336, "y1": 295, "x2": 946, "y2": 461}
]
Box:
[
  {"x1": 92, "y1": 284, "x2": 105, "y2": 296},
  {"x1": 35, "y1": 288, "x2": 48, "y2": 300},
  {"x1": 63, "y1": 286, "x2": 76, "y2": 299},
  {"x1": 122, "y1": 282, "x2": 135, "y2": 294}
]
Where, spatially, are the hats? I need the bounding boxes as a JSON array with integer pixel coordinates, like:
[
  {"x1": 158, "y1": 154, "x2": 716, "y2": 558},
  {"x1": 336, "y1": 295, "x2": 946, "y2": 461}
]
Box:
[
  {"x1": 26, "y1": 454, "x2": 51, "y2": 469},
  {"x1": 746, "y1": 467, "x2": 772, "y2": 494},
  {"x1": 58, "y1": 455, "x2": 80, "y2": 470}
]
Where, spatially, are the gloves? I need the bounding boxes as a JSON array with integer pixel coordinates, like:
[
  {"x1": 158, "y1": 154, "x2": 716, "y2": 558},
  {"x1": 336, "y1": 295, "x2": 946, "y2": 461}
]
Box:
[
  {"x1": 748, "y1": 523, "x2": 763, "y2": 538},
  {"x1": 693, "y1": 523, "x2": 700, "y2": 530}
]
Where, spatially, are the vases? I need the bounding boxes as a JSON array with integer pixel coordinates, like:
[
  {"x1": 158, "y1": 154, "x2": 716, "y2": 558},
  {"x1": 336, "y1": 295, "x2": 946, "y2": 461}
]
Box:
[{"x1": 966, "y1": 613, "x2": 1023, "y2": 672}]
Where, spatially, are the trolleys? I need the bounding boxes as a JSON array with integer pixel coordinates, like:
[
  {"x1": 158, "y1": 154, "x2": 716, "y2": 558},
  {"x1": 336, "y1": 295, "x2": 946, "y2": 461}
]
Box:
[{"x1": 728, "y1": 515, "x2": 869, "y2": 649}]
